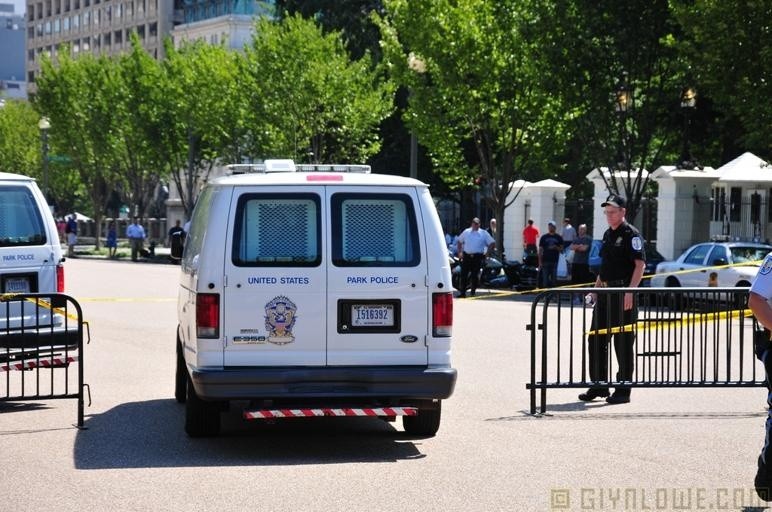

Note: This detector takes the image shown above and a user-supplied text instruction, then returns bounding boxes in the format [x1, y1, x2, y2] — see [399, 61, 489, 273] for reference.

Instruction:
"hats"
[601, 193, 626, 208]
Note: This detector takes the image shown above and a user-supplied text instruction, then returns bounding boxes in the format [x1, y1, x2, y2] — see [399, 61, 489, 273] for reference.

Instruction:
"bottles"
[584, 295, 597, 307]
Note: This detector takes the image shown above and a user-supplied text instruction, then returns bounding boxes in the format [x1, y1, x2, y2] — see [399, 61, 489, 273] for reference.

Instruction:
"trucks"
[170, 157, 458, 437]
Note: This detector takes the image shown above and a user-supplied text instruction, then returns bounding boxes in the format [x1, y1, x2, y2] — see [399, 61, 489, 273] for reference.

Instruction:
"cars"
[448, 252, 503, 291]
[650, 235, 772, 310]
[514, 238, 666, 298]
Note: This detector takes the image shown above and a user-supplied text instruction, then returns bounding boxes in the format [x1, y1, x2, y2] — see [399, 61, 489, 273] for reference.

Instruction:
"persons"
[56, 219, 68, 242]
[67, 213, 79, 258]
[125, 216, 146, 261]
[748, 252, 771, 501]
[106, 221, 118, 258]
[579, 194, 647, 405]
[443, 217, 592, 304]
[168, 219, 185, 264]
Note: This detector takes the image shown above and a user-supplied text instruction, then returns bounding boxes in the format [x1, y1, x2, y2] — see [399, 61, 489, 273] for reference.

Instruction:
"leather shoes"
[456, 293, 467, 298]
[606, 396, 630, 404]
[469, 291, 475, 297]
[755, 455, 772, 502]
[579, 389, 610, 401]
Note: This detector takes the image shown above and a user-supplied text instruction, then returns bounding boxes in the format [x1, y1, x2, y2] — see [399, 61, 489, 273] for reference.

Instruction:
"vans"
[1, 171, 84, 368]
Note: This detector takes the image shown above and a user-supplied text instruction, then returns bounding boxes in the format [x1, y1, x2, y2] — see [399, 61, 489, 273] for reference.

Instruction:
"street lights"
[38, 116, 52, 196]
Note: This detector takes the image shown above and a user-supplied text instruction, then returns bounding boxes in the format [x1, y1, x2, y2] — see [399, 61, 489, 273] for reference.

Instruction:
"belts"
[601, 279, 630, 287]
